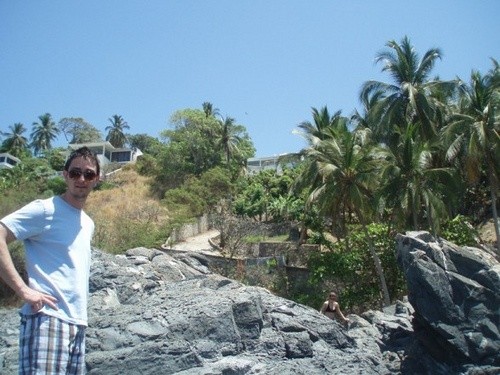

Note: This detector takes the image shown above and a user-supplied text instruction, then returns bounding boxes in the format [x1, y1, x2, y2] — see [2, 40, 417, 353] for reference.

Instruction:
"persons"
[319, 292, 350, 322]
[0, 145, 101, 375]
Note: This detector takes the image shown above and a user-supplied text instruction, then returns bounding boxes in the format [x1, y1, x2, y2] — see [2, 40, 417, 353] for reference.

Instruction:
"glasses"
[68, 169, 97, 181]
